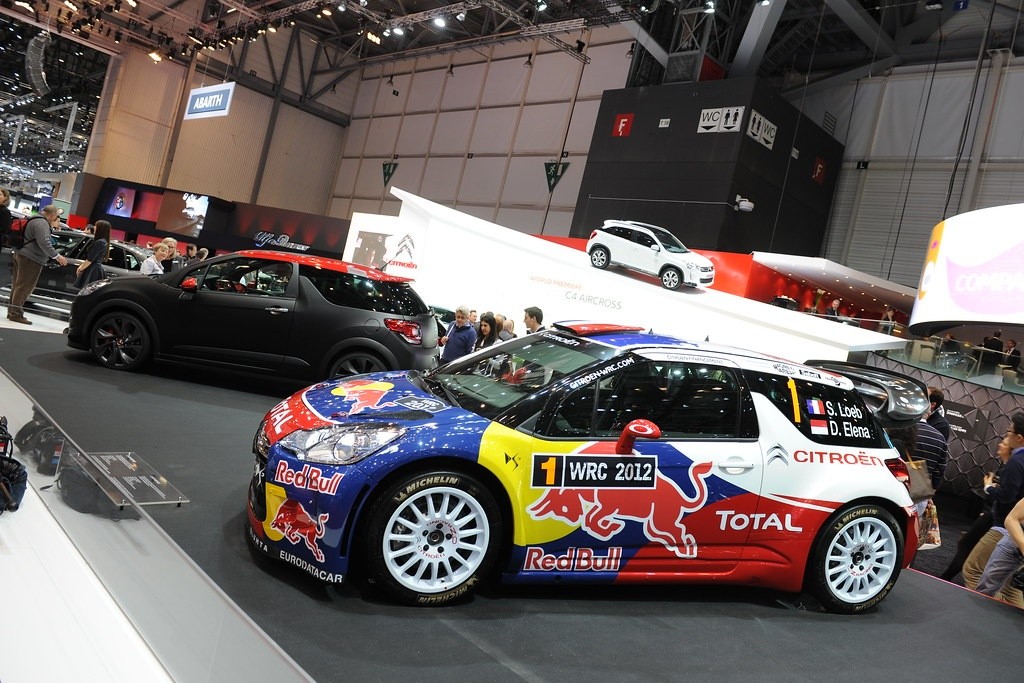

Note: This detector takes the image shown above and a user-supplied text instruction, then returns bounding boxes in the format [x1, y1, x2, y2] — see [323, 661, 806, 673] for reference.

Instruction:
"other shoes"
[7, 313, 32, 325]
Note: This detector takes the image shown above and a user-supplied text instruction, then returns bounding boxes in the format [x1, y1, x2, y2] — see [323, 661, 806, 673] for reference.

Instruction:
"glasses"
[1004, 429, 1017, 437]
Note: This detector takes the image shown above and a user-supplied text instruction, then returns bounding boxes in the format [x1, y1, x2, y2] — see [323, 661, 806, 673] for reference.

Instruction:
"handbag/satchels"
[905, 460, 935, 503]
[916, 501, 941, 552]
[439, 346, 445, 359]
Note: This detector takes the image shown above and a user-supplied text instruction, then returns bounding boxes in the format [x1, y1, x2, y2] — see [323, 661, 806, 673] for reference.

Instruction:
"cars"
[245, 321, 938, 614]
[61, 250, 439, 396]
[9, 209, 70, 235]
[7, 230, 155, 310]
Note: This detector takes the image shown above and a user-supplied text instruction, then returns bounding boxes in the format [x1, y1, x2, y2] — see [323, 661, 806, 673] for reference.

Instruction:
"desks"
[879, 323, 903, 334]
[837, 317, 860, 325]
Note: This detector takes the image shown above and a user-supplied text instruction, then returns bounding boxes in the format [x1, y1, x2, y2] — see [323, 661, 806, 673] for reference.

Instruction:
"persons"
[177, 249, 182, 256]
[184, 250, 207, 266]
[966, 337, 991, 372]
[523, 306, 546, 333]
[885, 386, 1024, 607]
[824, 299, 843, 322]
[480, 312, 493, 317]
[162, 237, 177, 273]
[130, 240, 134, 243]
[471, 314, 503, 354]
[1003, 339, 1021, 372]
[147, 242, 152, 248]
[84, 224, 94, 234]
[140, 243, 169, 274]
[480, 320, 514, 341]
[496, 316, 504, 333]
[52, 208, 64, 222]
[0, 186, 12, 258]
[469, 309, 479, 331]
[7, 205, 68, 325]
[200, 248, 209, 255]
[22, 206, 30, 214]
[975, 331, 1004, 375]
[72, 220, 111, 289]
[182, 244, 197, 262]
[939, 331, 962, 368]
[884, 307, 896, 339]
[440, 305, 477, 366]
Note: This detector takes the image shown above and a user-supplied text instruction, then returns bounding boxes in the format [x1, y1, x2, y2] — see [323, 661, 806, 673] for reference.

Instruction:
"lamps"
[0, 0, 770, 94]
[924, 0, 944, 11]
[735, 195, 754, 212]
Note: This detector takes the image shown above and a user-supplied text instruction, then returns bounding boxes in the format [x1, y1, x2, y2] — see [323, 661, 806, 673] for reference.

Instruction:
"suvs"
[584, 219, 717, 291]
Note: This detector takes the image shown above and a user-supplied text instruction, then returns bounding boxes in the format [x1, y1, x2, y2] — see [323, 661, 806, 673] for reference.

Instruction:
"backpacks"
[9, 215, 49, 250]
